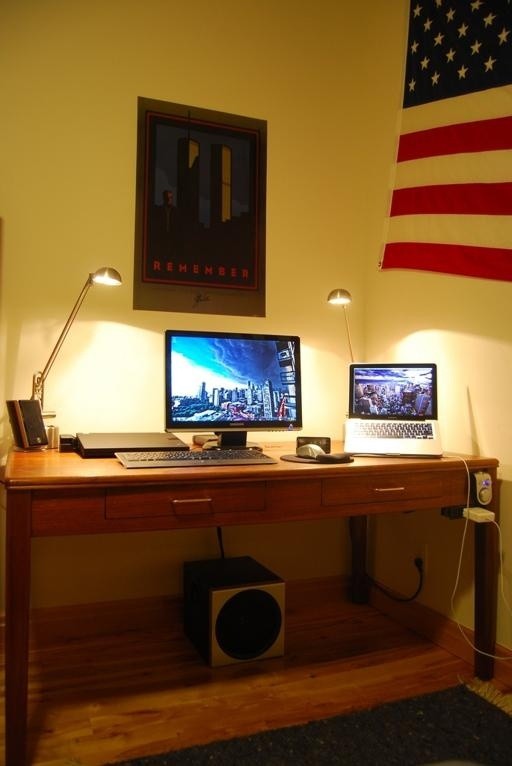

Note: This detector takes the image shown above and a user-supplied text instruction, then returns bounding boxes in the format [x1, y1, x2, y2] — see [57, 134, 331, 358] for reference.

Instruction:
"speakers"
[6, 398, 50, 452]
[183, 555, 287, 669]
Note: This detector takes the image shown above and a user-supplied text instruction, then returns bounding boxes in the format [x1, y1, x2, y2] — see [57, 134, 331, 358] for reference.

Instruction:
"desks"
[1, 437, 502, 766]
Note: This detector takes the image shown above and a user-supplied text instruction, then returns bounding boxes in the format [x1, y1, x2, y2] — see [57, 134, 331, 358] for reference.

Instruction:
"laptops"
[75, 431, 190, 459]
[343, 362, 445, 457]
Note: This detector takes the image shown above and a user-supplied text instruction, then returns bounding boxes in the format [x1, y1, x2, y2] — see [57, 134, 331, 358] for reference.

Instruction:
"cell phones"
[297, 437, 331, 453]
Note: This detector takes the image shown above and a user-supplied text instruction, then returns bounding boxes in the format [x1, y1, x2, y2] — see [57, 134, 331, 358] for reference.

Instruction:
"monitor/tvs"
[164, 329, 302, 451]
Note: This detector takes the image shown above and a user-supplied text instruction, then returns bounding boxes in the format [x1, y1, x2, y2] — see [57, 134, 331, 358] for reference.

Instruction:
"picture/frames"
[144, 111, 265, 294]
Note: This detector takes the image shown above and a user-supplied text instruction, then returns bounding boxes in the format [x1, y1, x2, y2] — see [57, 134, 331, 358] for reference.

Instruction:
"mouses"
[295, 444, 325, 459]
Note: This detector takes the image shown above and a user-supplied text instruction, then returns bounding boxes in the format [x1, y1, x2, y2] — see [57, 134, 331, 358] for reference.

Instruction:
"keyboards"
[114, 449, 279, 469]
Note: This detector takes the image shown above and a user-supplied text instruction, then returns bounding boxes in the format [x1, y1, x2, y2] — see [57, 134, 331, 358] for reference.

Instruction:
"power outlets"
[408, 543, 427, 577]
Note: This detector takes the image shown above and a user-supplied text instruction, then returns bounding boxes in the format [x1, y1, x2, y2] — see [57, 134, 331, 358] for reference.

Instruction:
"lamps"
[329, 287, 362, 364]
[38, 266, 127, 422]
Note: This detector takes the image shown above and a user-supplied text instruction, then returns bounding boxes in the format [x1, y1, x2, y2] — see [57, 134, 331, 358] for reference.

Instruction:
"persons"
[153, 188, 178, 243]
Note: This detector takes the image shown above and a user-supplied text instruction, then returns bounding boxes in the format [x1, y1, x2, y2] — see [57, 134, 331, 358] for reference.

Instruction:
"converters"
[463, 507, 497, 527]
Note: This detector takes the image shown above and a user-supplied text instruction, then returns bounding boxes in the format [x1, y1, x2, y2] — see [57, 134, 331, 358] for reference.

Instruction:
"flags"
[380, 0, 512, 285]
